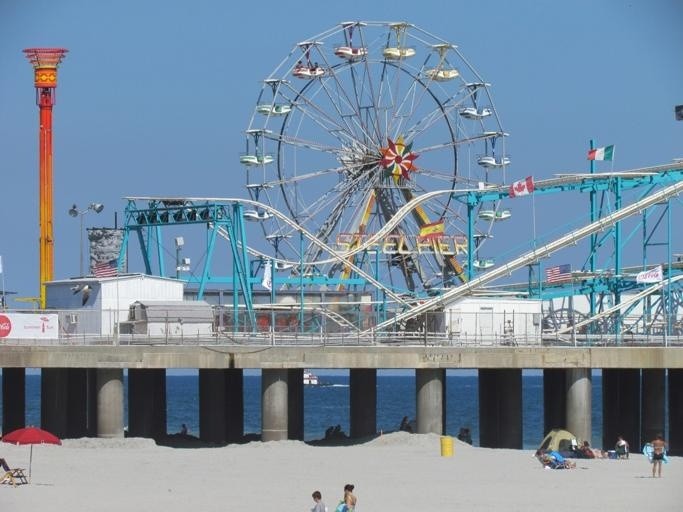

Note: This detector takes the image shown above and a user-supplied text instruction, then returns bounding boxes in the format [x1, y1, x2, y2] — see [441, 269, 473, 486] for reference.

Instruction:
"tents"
[536, 427, 579, 458]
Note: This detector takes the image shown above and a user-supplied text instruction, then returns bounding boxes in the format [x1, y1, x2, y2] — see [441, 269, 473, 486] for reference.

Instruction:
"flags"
[508, 174, 533, 198]
[545, 264, 572, 283]
[95, 258, 118, 278]
[261, 257, 272, 293]
[636, 264, 662, 284]
[418, 218, 445, 241]
[587, 145, 614, 161]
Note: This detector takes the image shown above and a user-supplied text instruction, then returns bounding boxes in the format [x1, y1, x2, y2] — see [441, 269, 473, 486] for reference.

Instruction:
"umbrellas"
[2, 425, 62, 482]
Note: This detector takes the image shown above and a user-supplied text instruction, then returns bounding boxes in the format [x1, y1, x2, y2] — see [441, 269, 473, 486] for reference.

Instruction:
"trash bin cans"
[440, 436, 454, 457]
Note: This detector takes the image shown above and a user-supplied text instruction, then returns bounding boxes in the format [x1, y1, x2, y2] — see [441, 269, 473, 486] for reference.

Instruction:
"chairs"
[0, 458, 29, 484]
[618, 444, 629, 460]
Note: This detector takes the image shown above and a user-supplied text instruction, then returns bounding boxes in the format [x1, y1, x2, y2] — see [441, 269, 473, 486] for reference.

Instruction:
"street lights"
[69, 201, 103, 279]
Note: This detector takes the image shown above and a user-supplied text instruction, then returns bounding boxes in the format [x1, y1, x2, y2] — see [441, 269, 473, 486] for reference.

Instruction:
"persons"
[400, 416, 411, 431]
[181, 424, 187, 435]
[324, 425, 350, 440]
[615, 435, 629, 458]
[457, 427, 473, 445]
[647, 433, 668, 478]
[311, 491, 326, 512]
[0, 457, 10, 472]
[344, 484, 357, 512]
